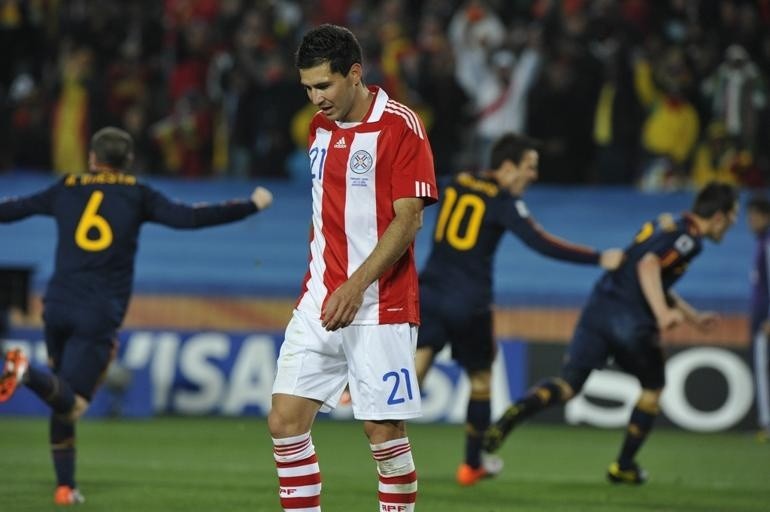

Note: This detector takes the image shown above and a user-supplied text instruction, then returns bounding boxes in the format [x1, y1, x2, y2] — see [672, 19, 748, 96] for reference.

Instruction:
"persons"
[483, 175, 737, 487]
[0, 127, 274, 505]
[411, 131, 624, 488]
[745, 197, 769, 430]
[268, 20, 442, 512]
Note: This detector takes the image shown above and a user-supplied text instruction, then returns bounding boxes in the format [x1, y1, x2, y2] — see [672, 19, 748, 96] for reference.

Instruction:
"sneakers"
[54, 483, 87, 506]
[605, 459, 651, 484]
[456, 448, 504, 486]
[2, 347, 31, 404]
